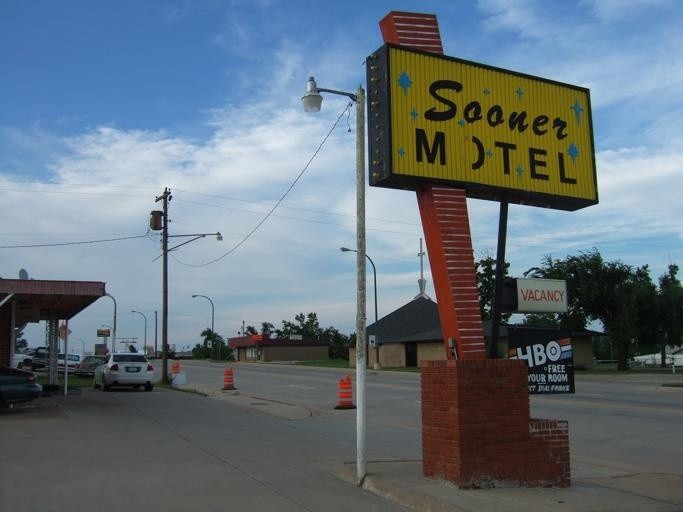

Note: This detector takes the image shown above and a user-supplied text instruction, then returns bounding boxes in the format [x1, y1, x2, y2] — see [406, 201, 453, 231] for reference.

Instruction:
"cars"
[12, 347, 105, 377]
[92, 352, 154, 392]
[0, 362, 42, 415]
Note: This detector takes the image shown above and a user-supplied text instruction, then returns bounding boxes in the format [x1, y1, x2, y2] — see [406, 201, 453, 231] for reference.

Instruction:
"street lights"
[99, 324, 113, 353]
[161, 231, 222, 385]
[103, 292, 116, 352]
[191, 295, 214, 360]
[130, 311, 146, 354]
[340, 247, 381, 369]
[75, 338, 84, 358]
[300, 76, 368, 485]
